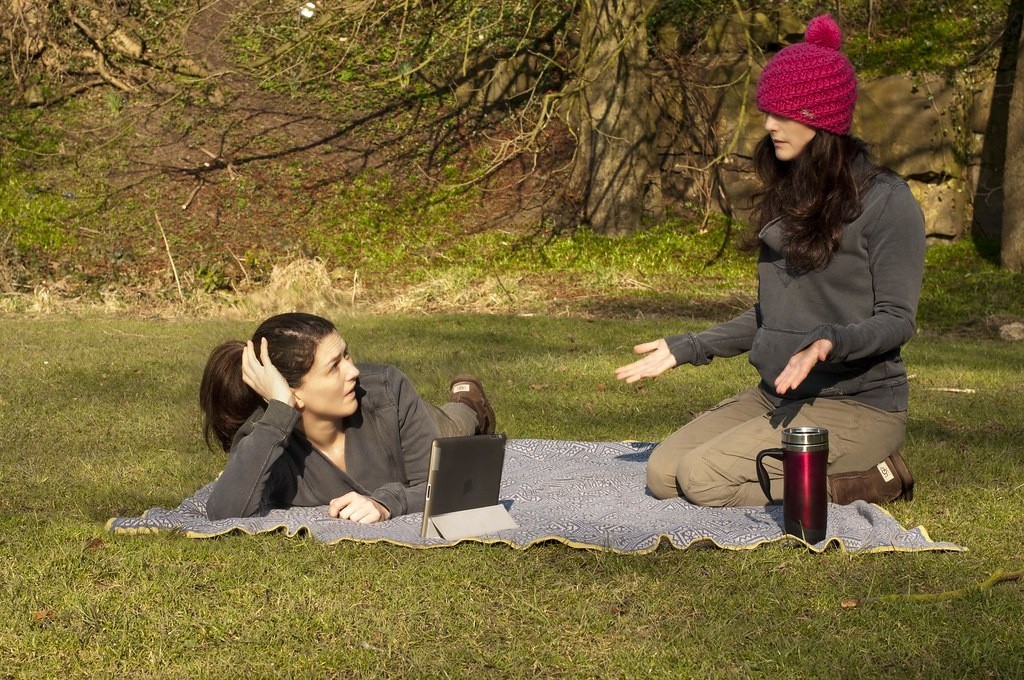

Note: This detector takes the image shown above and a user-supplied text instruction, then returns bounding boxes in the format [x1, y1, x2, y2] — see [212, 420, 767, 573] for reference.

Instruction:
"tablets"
[421, 433, 508, 538]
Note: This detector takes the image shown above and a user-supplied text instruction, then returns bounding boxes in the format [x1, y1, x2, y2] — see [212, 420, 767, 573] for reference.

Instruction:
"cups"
[756, 427, 830, 543]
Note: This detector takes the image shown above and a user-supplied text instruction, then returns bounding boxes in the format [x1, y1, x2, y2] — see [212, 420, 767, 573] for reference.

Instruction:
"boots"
[449, 375, 497, 436]
[828, 448, 913, 505]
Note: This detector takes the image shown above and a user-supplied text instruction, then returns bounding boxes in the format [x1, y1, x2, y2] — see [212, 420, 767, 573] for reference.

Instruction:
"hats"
[755, 15, 858, 135]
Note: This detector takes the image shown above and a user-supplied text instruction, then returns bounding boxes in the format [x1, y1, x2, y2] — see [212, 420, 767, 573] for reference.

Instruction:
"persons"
[616, 16, 927, 506]
[201, 313, 495, 523]
